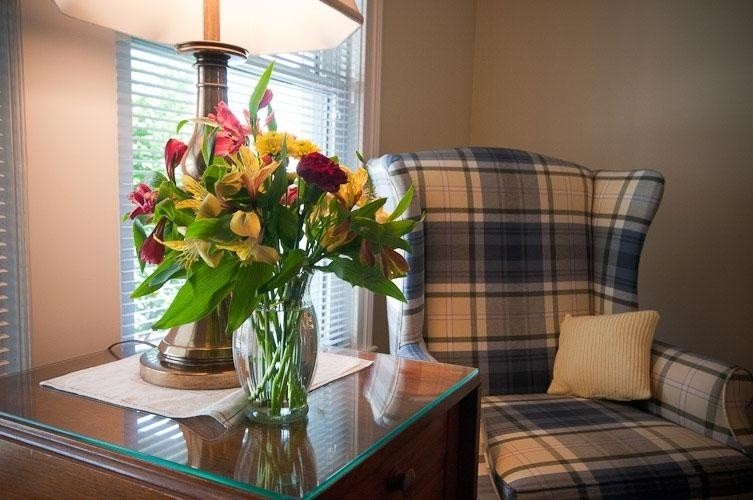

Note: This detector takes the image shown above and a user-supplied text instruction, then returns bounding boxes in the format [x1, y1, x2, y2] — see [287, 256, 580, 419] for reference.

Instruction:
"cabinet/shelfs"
[1, 333, 484, 499]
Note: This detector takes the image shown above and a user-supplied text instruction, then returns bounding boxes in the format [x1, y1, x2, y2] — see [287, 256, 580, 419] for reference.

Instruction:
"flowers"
[118, 54, 427, 419]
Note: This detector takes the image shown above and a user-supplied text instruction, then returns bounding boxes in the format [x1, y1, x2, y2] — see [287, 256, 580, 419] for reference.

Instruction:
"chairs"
[360, 140, 752, 500]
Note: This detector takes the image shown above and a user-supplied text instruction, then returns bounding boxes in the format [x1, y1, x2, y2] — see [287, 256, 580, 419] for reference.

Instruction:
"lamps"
[41, 1, 372, 398]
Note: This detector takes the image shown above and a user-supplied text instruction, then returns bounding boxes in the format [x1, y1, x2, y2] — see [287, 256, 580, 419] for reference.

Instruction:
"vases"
[222, 289, 328, 429]
[232, 420, 320, 499]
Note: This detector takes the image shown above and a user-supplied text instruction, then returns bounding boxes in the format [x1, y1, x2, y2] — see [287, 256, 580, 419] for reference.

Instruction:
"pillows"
[545, 308, 659, 401]
